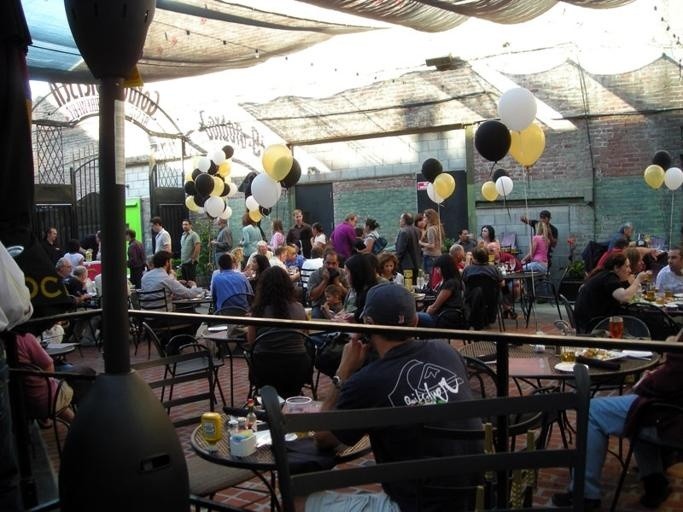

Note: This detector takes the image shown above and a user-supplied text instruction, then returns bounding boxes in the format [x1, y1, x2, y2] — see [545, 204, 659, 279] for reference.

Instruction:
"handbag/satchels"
[372, 238, 387, 254]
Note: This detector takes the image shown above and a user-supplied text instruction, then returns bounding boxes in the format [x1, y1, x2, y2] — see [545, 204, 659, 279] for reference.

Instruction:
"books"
[476, 86, 547, 205]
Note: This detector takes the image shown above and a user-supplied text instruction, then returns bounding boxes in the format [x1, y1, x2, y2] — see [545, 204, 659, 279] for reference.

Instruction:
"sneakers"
[552, 488, 602, 512]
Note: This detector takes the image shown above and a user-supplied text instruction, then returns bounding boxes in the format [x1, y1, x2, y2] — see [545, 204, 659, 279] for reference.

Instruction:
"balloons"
[421, 157, 456, 203]
[180, 141, 234, 220]
[643, 147, 683, 192]
[246, 141, 303, 223]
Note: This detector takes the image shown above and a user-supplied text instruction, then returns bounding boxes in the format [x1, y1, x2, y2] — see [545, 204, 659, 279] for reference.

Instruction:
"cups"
[304, 308, 312, 321]
[510, 247, 516, 254]
[634, 284, 673, 305]
[498, 257, 516, 275]
[559, 329, 576, 365]
[283, 396, 313, 441]
[609, 315, 622, 338]
[644, 233, 649, 242]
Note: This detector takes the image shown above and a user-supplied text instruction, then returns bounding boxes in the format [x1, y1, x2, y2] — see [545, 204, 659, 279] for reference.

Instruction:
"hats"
[359, 282, 416, 324]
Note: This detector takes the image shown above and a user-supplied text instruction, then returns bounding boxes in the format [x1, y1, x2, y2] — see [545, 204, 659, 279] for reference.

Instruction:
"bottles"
[40, 339, 48, 350]
[245, 399, 256, 433]
[534, 331, 545, 354]
[201, 411, 223, 452]
[237, 417, 246, 431]
[85, 250, 91, 261]
[416, 269, 424, 289]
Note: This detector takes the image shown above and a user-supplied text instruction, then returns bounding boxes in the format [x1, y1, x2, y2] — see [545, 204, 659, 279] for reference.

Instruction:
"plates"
[664, 303, 678, 309]
[576, 347, 628, 363]
[553, 362, 588, 372]
[673, 294, 682, 298]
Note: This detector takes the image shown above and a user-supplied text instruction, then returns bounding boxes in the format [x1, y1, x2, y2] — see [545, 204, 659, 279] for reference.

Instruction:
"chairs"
[258, 363, 591, 511]
[3, 362, 95, 508]
[208, 307, 250, 406]
[164, 342, 277, 511]
[604, 390, 681, 510]
[140, 321, 228, 407]
[0, 232, 682, 360]
[246, 329, 318, 402]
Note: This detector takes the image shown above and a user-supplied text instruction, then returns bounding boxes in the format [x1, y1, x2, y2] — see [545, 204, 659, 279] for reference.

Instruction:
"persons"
[573, 221, 683, 338]
[0, 238, 34, 492]
[551, 325, 683, 512]
[287, 283, 492, 511]
[13, 325, 76, 428]
[36, 209, 559, 397]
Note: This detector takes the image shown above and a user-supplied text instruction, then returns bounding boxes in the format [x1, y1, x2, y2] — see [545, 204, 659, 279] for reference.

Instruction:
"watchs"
[332, 374, 343, 388]
